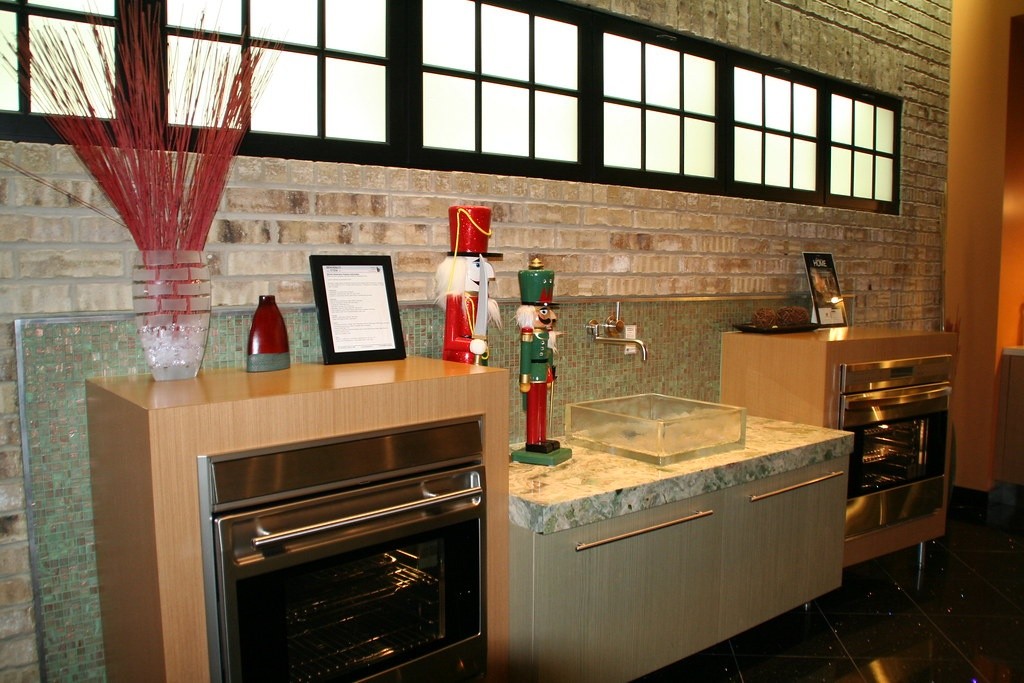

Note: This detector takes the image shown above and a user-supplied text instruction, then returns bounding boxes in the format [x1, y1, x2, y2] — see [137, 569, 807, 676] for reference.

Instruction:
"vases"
[132, 252, 212, 383]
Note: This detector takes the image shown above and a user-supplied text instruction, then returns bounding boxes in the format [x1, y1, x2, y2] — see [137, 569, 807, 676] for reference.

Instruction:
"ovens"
[720, 329, 958, 569]
[197, 417, 490, 683]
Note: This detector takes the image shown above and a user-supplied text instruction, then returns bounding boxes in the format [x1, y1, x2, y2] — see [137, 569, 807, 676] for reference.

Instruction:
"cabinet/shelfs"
[508, 431, 855, 683]
